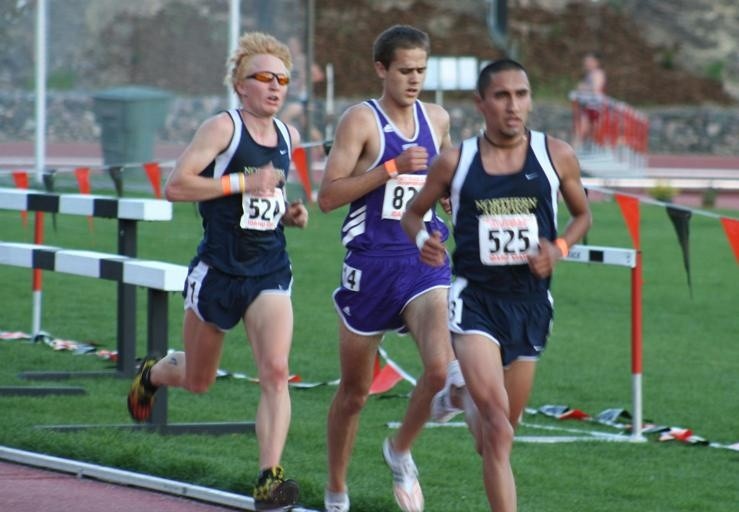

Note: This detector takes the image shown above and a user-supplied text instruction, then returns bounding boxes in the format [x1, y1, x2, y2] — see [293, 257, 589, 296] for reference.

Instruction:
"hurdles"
[383, 243, 647, 445]
[3, 241, 258, 436]
[1, 188, 176, 382]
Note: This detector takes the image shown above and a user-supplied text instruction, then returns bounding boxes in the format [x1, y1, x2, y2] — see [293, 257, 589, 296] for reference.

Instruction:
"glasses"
[240, 71, 292, 86]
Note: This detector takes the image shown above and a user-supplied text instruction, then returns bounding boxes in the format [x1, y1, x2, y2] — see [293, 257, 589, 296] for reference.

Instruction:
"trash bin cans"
[90, 85, 176, 186]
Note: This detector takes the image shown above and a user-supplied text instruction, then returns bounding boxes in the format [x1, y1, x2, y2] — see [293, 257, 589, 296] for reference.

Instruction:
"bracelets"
[555, 239, 573, 262]
[384, 159, 397, 178]
[411, 228, 432, 255]
[220, 171, 246, 195]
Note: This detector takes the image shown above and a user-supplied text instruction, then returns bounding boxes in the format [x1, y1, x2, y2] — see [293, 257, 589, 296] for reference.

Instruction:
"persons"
[316, 23, 453, 512]
[572, 53, 608, 155]
[398, 58, 593, 510]
[282, 37, 324, 159]
[126, 29, 309, 512]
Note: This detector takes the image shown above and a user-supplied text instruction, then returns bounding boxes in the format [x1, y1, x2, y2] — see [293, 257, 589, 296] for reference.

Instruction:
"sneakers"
[126, 350, 158, 423]
[253, 465, 300, 512]
[381, 434, 425, 511]
[428, 358, 467, 424]
[323, 483, 350, 512]
